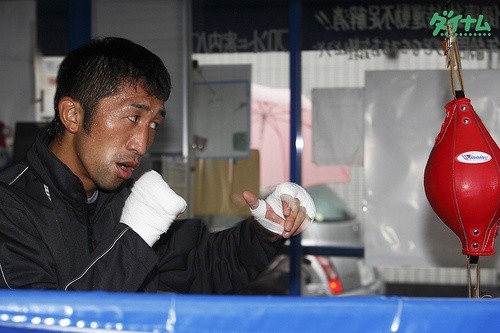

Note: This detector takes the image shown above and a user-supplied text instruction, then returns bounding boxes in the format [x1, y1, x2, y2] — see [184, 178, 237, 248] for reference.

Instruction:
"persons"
[0, 34, 318, 296]
